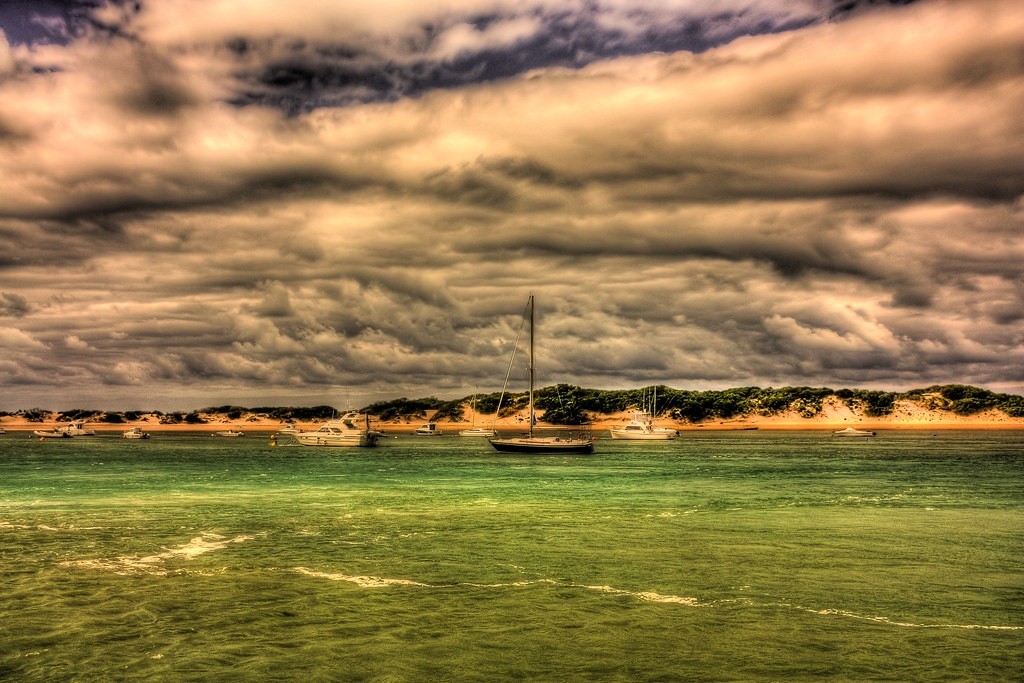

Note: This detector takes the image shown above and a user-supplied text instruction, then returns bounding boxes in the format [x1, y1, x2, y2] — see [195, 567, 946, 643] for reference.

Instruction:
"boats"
[278, 424, 304, 435]
[216, 430, 244, 437]
[296, 412, 387, 449]
[32, 427, 73, 438]
[416, 422, 444, 439]
[61, 422, 97, 436]
[835, 427, 876, 439]
[122, 427, 151, 439]
[607, 384, 679, 441]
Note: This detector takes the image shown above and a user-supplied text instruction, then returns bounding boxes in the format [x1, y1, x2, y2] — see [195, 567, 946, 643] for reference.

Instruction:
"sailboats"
[487, 291, 595, 454]
[458, 384, 500, 438]
